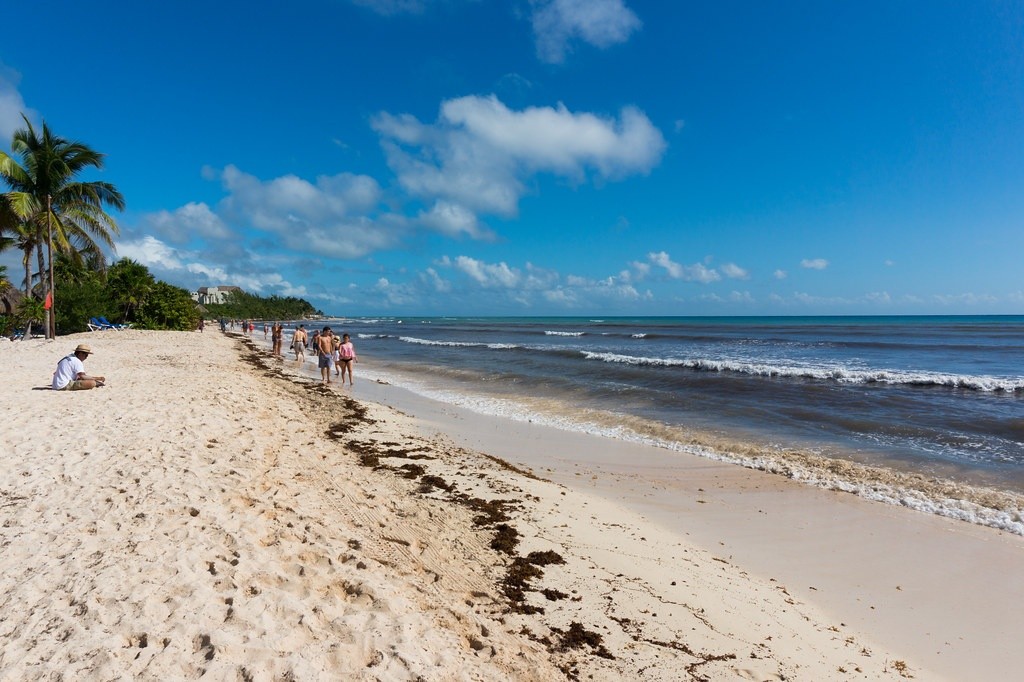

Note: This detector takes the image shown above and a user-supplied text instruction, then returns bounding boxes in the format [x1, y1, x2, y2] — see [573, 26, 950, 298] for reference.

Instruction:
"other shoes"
[95, 380, 105, 387]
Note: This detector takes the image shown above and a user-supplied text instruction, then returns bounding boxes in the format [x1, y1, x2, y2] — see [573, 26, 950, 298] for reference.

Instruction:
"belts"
[295, 341, 304, 342]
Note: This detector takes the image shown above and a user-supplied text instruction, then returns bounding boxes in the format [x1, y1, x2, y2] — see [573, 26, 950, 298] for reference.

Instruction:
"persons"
[199, 319, 205, 333]
[51, 344, 106, 391]
[231, 318, 234, 327]
[242, 320, 252, 334]
[271, 323, 283, 355]
[332, 336, 341, 376]
[288, 322, 290, 326]
[220, 318, 228, 331]
[329, 331, 334, 338]
[291, 326, 307, 362]
[300, 324, 307, 337]
[310, 330, 320, 356]
[317, 327, 332, 383]
[264, 323, 268, 340]
[338, 334, 358, 385]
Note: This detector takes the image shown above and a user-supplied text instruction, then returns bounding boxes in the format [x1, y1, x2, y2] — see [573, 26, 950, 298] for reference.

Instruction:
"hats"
[332, 336, 341, 342]
[73, 344, 93, 354]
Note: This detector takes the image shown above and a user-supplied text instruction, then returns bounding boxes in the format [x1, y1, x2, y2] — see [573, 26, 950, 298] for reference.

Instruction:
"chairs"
[99, 317, 134, 332]
[87, 317, 120, 335]
